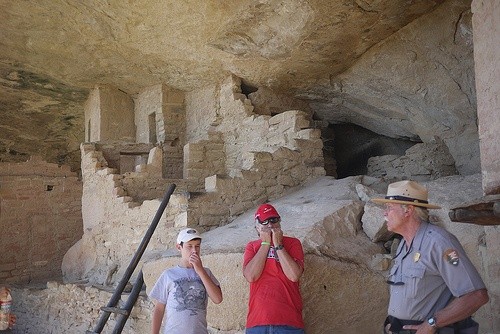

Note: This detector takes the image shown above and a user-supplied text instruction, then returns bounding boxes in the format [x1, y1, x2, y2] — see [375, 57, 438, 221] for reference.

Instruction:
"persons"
[0, 285, 16, 334]
[148, 228, 223, 334]
[242, 203, 305, 334]
[372, 181, 489, 334]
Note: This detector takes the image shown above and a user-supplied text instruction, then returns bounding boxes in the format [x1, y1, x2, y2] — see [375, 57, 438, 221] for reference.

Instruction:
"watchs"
[427, 316, 438, 331]
[275, 245, 284, 251]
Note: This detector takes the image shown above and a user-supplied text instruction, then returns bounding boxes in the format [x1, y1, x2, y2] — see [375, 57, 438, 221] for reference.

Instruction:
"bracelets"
[261, 241, 271, 245]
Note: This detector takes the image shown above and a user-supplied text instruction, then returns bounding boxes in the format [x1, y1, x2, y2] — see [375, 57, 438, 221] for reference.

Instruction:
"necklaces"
[387, 236, 415, 285]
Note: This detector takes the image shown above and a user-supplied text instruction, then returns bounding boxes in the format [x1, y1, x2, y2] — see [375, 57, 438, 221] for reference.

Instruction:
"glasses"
[386, 260, 404, 286]
[257, 217, 278, 225]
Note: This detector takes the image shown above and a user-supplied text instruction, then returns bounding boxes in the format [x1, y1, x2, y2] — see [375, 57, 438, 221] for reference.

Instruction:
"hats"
[371, 180, 442, 209]
[255, 204, 280, 221]
[177, 228, 202, 244]
